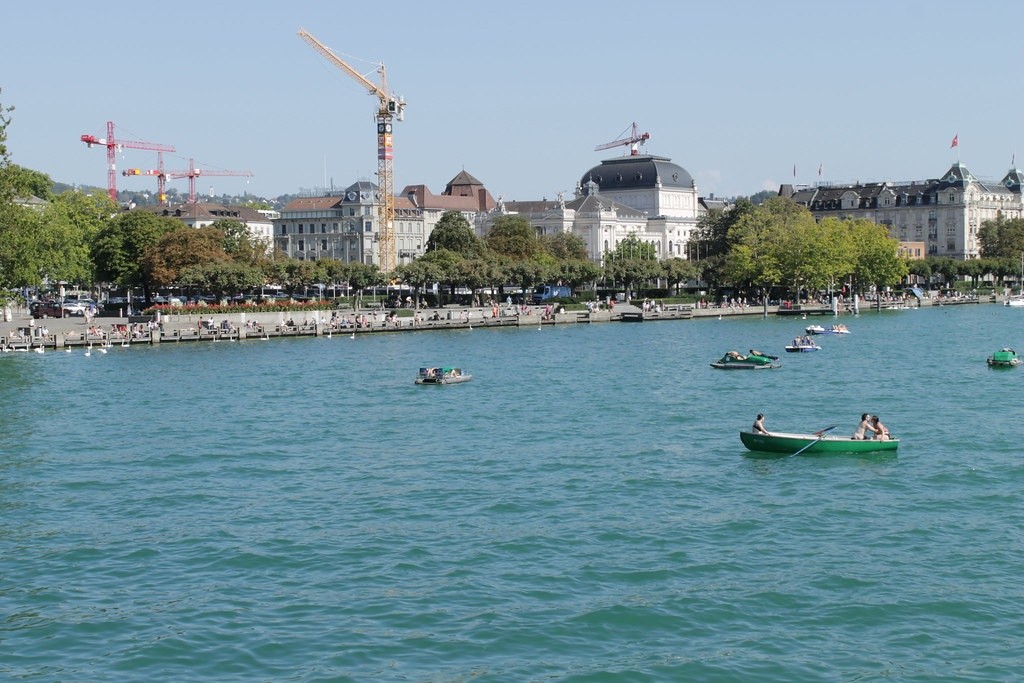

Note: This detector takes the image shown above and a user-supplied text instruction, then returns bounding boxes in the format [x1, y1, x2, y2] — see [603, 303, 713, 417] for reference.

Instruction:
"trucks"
[531, 283, 571, 305]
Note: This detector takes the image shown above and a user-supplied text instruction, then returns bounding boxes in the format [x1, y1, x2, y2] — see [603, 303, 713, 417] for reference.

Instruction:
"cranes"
[298, 27, 408, 290]
[172, 156, 256, 204]
[81, 120, 177, 207]
[119, 151, 205, 207]
[593, 121, 649, 158]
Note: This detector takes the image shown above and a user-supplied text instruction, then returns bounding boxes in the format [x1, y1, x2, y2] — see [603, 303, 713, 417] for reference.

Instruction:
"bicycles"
[394, 299, 403, 308]
[404, 301, 415, 311]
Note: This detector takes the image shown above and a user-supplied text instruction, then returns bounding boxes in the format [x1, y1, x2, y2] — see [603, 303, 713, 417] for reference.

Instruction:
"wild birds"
[0, 343, 45, 354]
[940, 303, 943, 307]
[718, 315, 722, 320]
[120, 339, 130, 348]
[229, 336, 235, 342]
[212, 335, 221, 343]
[327, 331, 333, 339]
[65, 346, 72, 353]
[259, 334, 270, 341]
[537, 326, 542, 331]
[802, 314, 807, 320]
[83, 340, 114, 357]
[349, 333, 356, 340]
[469, 324, 474, 330]
[885, 304, 918, 310]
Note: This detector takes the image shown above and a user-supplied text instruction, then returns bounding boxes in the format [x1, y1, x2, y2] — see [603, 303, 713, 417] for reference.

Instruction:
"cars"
[55, 283, 323, 317]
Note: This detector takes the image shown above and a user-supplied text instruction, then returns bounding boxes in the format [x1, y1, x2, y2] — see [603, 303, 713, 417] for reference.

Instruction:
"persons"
[753, 413, 770, 435]
[749, 350, 778, 360]
[8, 269, 1021, 349]
[871, 416, 889, 441]
[853, 413, 879, 440]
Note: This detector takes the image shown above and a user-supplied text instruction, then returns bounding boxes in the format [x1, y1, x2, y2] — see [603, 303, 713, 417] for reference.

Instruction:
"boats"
[805, 325, 851, 336]
[987, 347, 1023, 367]
[415, 364, 472, 385]
[710, 353, 782, 371]
[740, 429, 901, 453]
[1003, 294, 1024, 306]
[785, 339, 822, 353]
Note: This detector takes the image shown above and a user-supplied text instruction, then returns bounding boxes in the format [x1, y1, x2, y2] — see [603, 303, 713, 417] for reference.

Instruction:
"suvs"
[29, 301, 71, 320]
